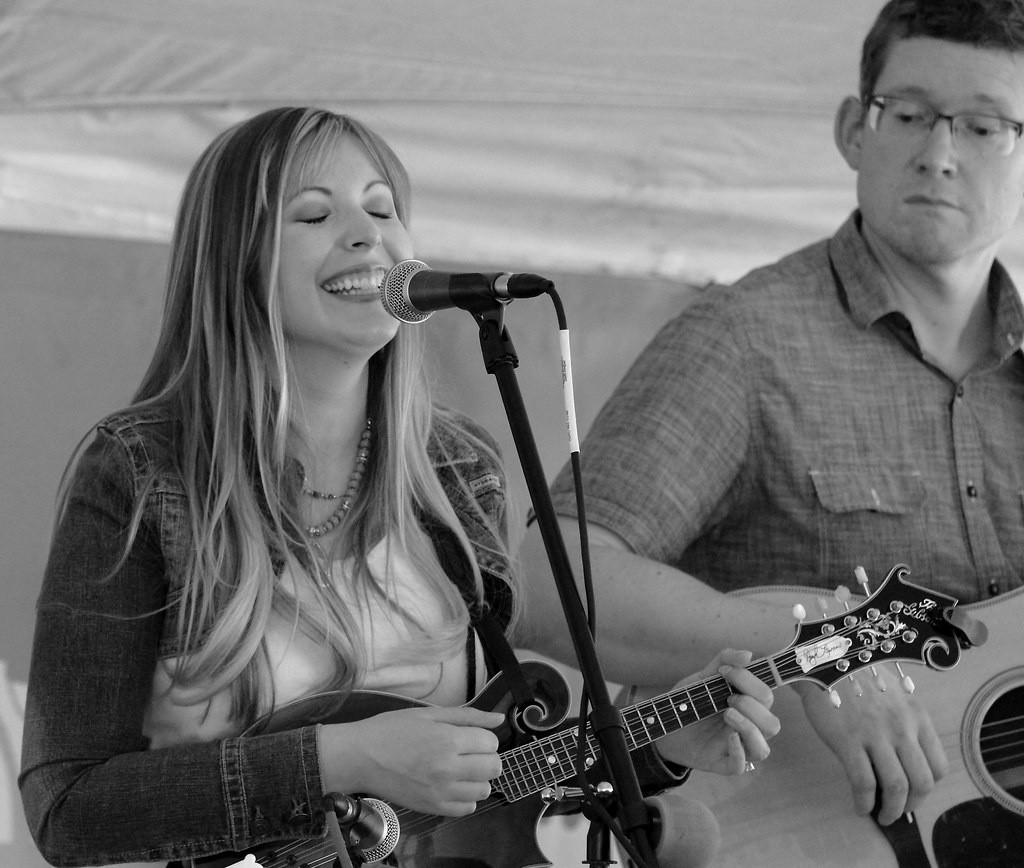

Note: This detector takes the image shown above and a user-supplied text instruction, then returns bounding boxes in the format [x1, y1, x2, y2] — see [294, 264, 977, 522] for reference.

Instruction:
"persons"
[518, 0, 1024, 868]
[17, 105, 778, 868]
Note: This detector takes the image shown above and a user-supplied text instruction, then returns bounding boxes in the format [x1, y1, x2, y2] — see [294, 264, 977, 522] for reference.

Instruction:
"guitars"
[167, 568, 987, 868]
[605, 583, 1023, 867]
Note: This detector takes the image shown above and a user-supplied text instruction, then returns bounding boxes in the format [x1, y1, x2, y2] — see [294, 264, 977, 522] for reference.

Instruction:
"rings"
[745, 760, 756, 778]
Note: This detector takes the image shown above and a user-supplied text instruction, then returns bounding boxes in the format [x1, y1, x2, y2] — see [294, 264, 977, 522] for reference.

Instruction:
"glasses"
[860, 93, 1024, 162]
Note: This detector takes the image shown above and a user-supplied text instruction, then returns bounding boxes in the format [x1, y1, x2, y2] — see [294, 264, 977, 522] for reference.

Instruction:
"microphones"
[380, 259, 555, 324]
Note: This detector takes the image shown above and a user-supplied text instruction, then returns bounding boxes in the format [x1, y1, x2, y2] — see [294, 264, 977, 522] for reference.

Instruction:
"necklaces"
[298, 400, 377, 537]
